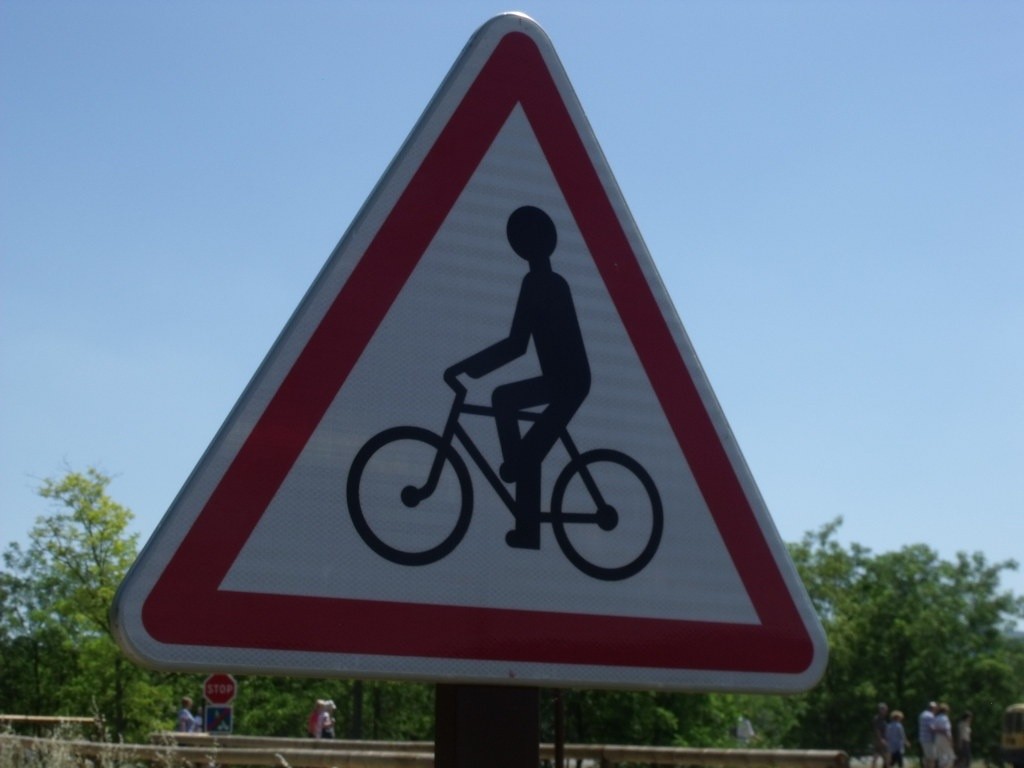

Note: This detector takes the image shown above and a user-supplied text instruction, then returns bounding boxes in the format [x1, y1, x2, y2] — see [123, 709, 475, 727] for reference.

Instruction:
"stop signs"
[204, 674, 237, 704]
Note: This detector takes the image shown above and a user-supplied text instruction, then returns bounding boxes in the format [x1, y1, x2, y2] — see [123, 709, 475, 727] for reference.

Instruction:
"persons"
[918, 701, 972, 768]
[736, 712, 761, 743]
[308, 699, 336, 738]
[871, 702, 911, 768]
[176, 696, 203, 732]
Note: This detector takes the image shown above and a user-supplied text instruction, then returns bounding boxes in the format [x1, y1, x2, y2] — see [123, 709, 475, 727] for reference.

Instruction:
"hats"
[324, 700, 337, 710]
